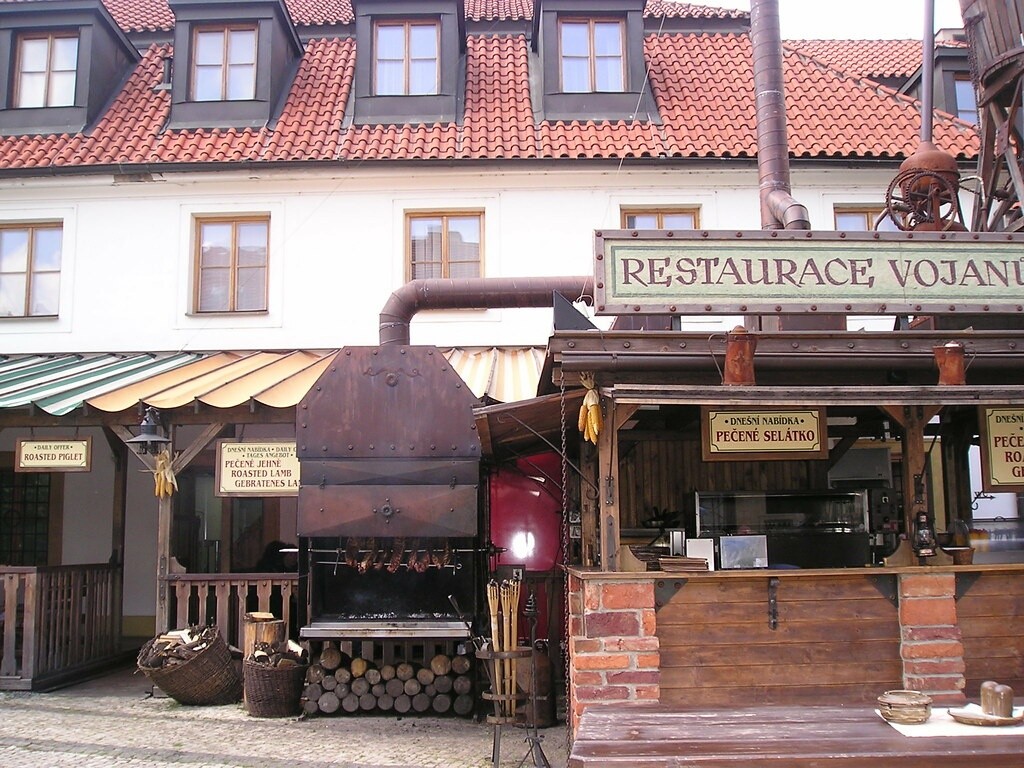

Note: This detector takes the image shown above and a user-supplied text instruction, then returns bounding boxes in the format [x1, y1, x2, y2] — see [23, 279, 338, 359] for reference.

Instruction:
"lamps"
[124, 406, 171, 458]
[912, 507, 938, 557]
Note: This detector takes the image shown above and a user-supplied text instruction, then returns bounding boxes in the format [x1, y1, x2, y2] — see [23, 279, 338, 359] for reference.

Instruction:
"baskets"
[138, 624, 243, 706]
[243, 654, 308, 717]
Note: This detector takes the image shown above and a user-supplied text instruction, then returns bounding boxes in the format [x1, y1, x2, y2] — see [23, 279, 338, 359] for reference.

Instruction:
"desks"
[566, 697, 1024, 768]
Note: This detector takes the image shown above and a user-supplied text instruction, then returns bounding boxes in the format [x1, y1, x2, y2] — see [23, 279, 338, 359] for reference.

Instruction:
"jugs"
[708, 325, 760, 386]
[932, 339, 977, 386]
